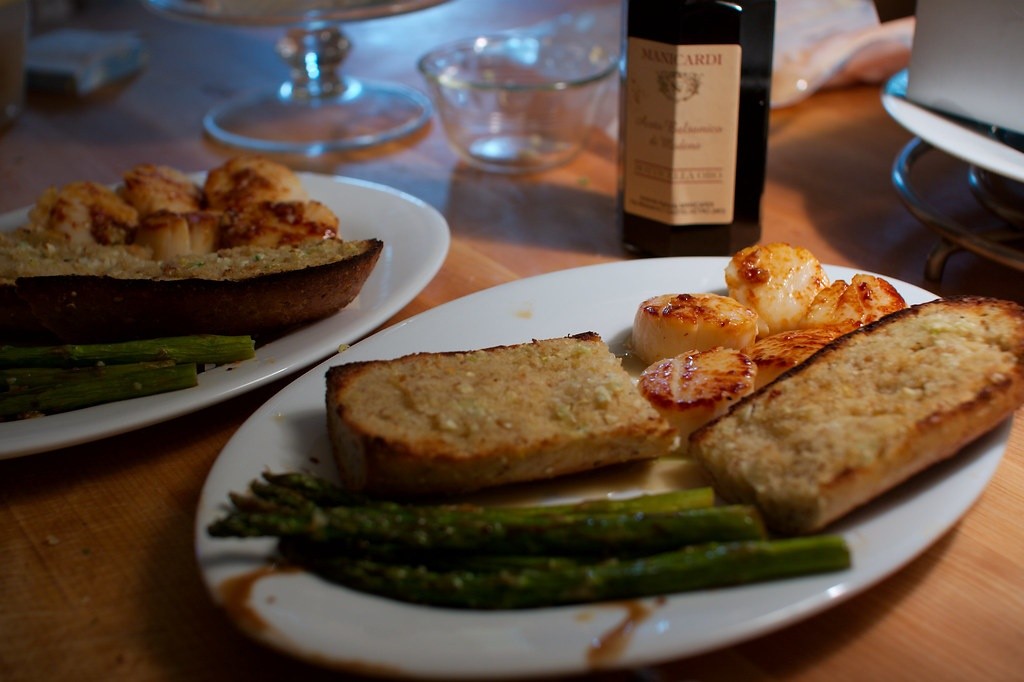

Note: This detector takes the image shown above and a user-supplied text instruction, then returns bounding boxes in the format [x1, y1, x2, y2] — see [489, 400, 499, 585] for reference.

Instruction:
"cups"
[0, 0, 28, 135]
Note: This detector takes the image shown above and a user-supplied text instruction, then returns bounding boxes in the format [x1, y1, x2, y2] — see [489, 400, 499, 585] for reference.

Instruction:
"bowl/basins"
[416, 32, 624, 174]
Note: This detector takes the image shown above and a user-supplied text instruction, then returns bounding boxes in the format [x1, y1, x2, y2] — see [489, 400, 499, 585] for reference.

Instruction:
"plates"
[0, 170, 450, 461]
[194, 256, 1015, 678]
[878, 67, 1024, 184]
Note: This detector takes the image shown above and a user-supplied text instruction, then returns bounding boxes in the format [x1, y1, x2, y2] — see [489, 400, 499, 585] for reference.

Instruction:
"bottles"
[626, 0, 777, 260]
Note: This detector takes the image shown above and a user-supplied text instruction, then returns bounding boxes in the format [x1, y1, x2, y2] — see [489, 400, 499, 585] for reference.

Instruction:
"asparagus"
[205, 470, 851, 612]
[0, 333, 256, 422]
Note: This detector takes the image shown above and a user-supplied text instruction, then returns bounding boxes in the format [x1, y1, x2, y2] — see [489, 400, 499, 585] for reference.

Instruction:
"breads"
[324, 331, 681, 497]
[1, 233, 383, 335]
[679, 290, 1023, 535]
